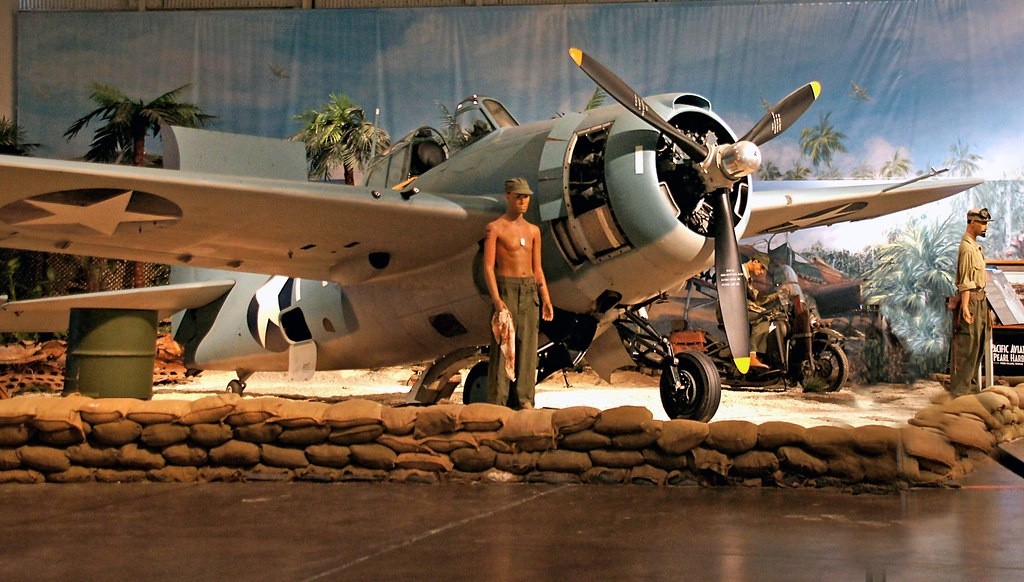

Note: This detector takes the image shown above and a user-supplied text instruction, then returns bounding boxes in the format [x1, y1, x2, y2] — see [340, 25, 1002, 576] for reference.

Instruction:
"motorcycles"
[704, 264, 848, 392]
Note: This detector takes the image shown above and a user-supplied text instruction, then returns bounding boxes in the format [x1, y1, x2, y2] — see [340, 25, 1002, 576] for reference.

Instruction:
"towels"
[491, 307, 520, 382]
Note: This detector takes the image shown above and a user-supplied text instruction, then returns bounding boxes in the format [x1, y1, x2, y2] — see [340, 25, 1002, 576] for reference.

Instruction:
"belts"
[969, 291, 987, 300]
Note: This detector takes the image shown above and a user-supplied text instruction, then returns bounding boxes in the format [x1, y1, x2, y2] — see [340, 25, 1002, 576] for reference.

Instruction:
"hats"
[504, 177, 534, 195]
[966, 208, 995, 222]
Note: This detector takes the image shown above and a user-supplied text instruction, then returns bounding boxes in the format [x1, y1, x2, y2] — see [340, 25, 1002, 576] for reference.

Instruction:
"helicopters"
[1, 46, 984, 423]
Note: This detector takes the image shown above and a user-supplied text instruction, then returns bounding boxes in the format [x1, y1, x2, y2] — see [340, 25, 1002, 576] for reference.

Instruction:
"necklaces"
[520, 237, 527, 247]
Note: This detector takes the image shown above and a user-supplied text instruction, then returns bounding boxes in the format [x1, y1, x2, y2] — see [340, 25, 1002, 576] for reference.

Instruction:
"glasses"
[968, 208, 992, 219]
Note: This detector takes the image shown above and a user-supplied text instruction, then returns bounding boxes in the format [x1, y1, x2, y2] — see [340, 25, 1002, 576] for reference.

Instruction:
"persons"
[481, 177, 554, 411]
[948, 207, 997, 398]
[716, 251, 773, 373]
